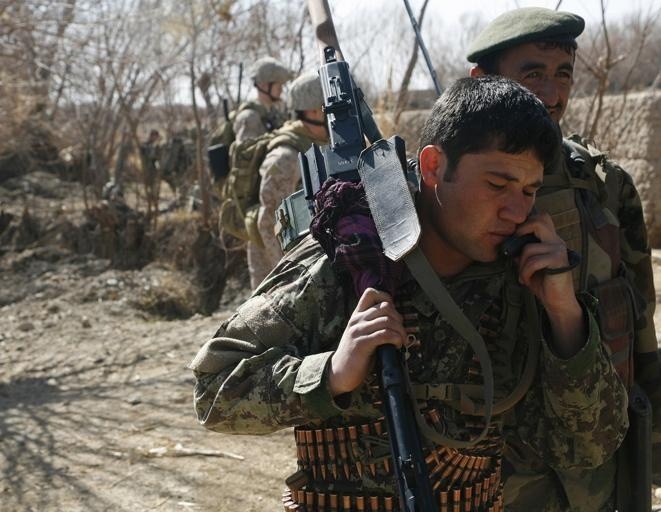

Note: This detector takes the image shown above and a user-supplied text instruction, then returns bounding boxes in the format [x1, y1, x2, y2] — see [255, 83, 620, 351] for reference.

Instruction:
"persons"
[140, 130, 160, 179]
[190, 75, 630, 512]
[208, 57, 329, 292]
[465, 7, 661, 512]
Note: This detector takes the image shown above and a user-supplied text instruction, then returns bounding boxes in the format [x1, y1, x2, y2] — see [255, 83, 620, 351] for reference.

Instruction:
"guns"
[298, 0, 433, 512]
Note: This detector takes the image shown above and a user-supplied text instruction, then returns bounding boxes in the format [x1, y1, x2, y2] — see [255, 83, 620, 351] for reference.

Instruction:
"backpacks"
[215, 131, 314, 250]
[206, 99, 272, 201]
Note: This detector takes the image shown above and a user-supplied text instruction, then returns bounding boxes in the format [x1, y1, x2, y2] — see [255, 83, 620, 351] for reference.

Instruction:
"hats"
[459, 4, 587, 64]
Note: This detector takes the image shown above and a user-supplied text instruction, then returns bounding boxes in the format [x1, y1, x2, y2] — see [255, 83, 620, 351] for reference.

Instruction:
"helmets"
[248, 59, 291, 86]
[286, 69, 325, 112]
